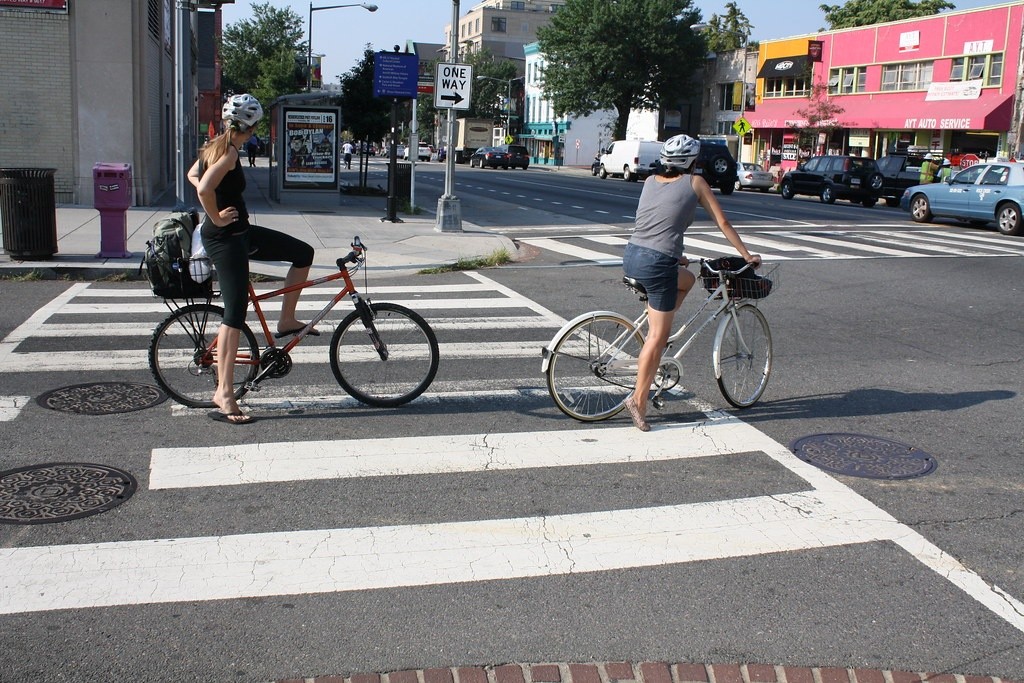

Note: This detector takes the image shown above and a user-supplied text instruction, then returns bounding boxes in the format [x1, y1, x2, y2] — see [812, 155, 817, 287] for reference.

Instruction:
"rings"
[234, 219, 236, 222]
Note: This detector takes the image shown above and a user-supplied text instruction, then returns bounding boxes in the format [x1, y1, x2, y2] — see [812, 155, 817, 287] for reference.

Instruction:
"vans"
[598, 139, 666, 182]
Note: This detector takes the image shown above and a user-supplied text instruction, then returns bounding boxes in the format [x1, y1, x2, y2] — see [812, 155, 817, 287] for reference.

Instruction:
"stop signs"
[959, 154, 981, 173]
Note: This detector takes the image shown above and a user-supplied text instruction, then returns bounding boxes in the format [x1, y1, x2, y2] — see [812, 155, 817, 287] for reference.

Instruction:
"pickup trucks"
[870, 154, 949, 208]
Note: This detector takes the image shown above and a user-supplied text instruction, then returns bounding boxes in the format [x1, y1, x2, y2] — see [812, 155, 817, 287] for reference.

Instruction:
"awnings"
[756, 54, 813, 80]
[737, 94, 1013, 132]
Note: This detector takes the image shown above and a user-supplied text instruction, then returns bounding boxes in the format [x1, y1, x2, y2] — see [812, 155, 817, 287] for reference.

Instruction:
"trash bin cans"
[0, 168, 59, 261]
[386, 163, 418, 209]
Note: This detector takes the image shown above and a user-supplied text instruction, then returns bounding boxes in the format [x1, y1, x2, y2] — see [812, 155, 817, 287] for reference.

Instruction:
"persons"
[342, 141, 353, 170]
[920, 153, 939, 184]
[288, 135, 316, 169]
[622, 134, 762, 432]
[246, 135, 257, 167]
[937, 159, 955, 182]
[188, 93, 320, 423]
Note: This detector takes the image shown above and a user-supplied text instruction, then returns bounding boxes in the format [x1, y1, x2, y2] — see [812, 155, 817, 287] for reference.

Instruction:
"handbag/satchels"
[247, 144, 258, 152]
[188, 223, 211, 284]
[701, 257, 772, 300]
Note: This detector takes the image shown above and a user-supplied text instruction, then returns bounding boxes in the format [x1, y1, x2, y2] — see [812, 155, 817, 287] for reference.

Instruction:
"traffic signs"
[434, 62, 474, 113]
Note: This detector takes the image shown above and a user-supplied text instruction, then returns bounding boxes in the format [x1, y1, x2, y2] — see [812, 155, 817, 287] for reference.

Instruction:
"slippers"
[208, 412, 254, 424]
[274, 324, 321, 336]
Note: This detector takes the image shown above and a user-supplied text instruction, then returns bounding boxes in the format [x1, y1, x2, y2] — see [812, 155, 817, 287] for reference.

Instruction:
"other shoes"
[252, 163, 256, 167]
[348, 166, 350, 169]
[345, 165, 347, 169]
[623, 398, 650, 432]
[250, 163, 252, 167]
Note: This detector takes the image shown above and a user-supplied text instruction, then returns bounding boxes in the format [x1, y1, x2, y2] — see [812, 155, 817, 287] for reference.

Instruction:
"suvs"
[499, 144, 532, 170]
[403, 142, 432, 161]
[654, 142, 738, 195]
[779, 155, 883, 207]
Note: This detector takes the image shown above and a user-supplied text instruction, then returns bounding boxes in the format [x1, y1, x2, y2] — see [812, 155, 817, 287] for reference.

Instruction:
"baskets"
[699, 263, 781, 301]
[143, 258, 212, 297]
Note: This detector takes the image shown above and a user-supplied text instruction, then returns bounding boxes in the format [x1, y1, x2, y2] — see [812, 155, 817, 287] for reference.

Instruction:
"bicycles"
[145, 235, 440, 408]
[540, 254, 775, 420]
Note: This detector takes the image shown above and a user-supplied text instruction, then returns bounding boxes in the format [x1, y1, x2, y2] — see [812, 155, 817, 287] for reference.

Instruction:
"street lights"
[691, 22, 752, 166]
[307, 1, 381, 92]
[476, 74, 525, 143]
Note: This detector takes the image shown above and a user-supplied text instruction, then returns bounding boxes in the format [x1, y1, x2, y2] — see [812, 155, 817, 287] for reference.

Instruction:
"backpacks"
[138, 208, 198, 296]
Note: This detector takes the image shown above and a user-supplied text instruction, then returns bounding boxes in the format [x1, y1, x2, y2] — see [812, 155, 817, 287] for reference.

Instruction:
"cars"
[589, 148, 604, 175]
[901, 155, 1024, 237]
[736, 162, 777, 192]
[356, 144, 376, 156]
[386, 145, 405, 159]
[469, 146, 510, 171]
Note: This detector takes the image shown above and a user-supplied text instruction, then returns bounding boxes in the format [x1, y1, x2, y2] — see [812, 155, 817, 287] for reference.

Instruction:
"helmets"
[659, 134, 700, 168]
[221, 94, 263, 127]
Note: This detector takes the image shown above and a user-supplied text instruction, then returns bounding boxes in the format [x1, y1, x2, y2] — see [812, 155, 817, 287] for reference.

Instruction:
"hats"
[924, 153, 932, 160]
[943, 159, 951, 165]
